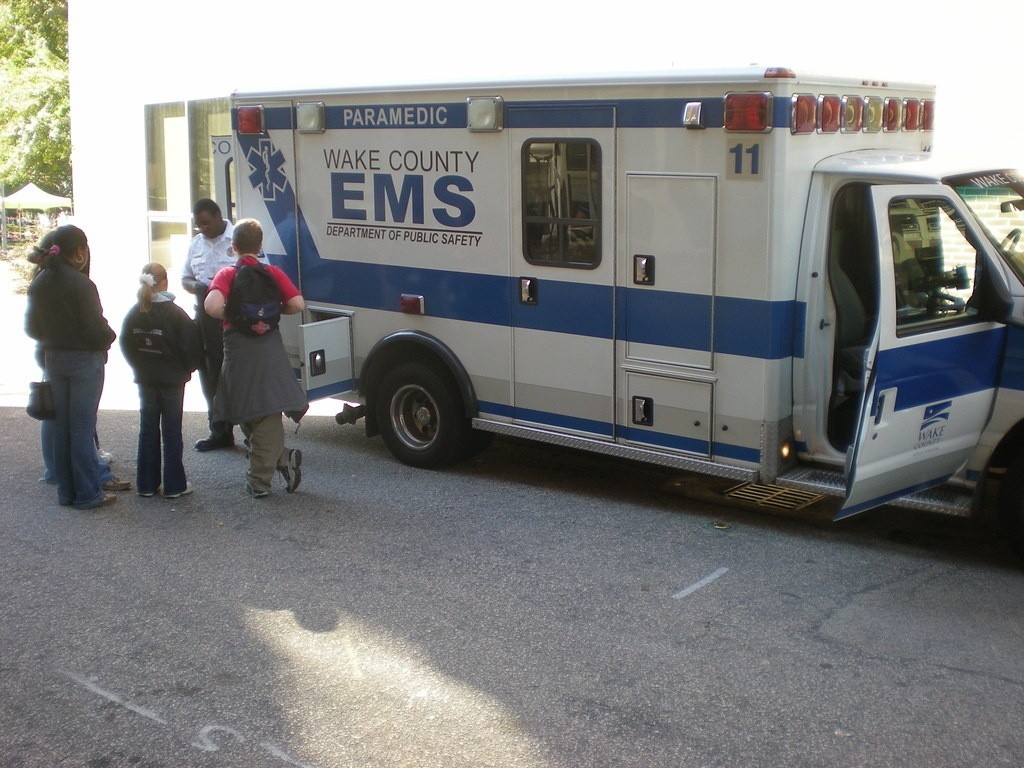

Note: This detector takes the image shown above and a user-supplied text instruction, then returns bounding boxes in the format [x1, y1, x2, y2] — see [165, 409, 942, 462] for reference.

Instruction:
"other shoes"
[246, 482, 268, 498]
[97, 448, 113, 463]
[101, 477, 131, 491]
[137, 485, 161, 496]
[194, 438, 234, 450]
[165, 481, 192, 498]
[96, 494, 117, 507]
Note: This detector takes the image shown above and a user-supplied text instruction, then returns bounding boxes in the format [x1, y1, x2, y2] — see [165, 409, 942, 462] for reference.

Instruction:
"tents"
[0, 183, 72, 240]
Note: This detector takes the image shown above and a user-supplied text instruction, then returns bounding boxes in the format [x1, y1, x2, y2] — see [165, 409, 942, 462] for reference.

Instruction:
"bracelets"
[193, 281, 199, 290]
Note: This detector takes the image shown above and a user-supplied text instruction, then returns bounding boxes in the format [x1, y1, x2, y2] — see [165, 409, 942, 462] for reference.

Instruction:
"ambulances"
[210, 64, 1024, 574]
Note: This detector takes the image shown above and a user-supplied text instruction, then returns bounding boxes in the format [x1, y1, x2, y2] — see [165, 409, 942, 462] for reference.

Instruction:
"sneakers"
[276, 448, 302, 493]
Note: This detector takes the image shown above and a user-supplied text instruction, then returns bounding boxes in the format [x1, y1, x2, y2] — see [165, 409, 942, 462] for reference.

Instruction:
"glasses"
[193, 217, 215, 234]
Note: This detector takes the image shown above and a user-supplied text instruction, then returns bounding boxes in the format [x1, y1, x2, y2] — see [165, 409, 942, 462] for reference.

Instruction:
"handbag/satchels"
[26, 381, 55, 421]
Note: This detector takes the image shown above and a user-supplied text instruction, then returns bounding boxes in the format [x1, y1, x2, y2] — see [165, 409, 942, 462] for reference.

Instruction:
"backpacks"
[228, 263, 281, 336]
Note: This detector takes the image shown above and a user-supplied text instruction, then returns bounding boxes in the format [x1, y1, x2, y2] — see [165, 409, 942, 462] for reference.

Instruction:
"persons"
[566, 210, 596, 263]
[182, 199, 270, 458]
[119, 263, 204, 500]
[204, 218, 305, 497]
[27, 224, 130, 508]
